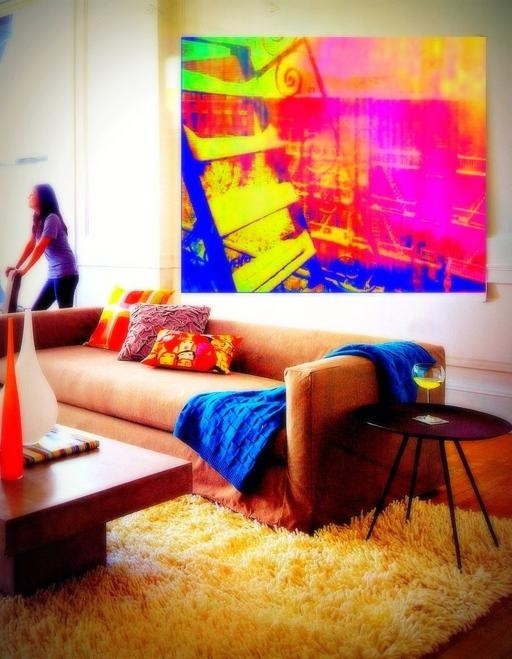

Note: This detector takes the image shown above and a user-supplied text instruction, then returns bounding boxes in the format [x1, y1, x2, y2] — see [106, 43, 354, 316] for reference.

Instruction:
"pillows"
[139, 324, 246, 375]
[80, 280, 177, 355]
[114, 300, 212, 364]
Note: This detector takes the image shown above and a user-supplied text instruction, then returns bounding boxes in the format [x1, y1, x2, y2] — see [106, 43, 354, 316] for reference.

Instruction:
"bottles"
[0, 309, 60, 448]
[1, 316, 26, 481]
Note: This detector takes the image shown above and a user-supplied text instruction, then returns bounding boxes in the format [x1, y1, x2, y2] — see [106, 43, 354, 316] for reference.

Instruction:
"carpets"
[0, 492, 512, 658]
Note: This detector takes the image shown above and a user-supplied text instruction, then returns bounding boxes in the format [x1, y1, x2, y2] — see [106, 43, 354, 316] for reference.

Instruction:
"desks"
[344, 395, 510, 572]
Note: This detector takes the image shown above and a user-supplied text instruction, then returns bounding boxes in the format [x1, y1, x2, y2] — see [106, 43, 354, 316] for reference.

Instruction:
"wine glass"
[411, 362, 451, 425]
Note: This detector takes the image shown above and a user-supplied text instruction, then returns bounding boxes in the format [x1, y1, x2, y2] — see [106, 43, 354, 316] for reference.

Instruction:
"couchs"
[1, 298, 454, 535]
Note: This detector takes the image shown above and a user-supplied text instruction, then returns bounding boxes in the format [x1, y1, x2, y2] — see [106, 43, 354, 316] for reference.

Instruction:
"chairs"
[0, 269, 22, 313]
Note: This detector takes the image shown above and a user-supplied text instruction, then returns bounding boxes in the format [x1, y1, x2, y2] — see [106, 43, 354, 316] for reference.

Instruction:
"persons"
[4, 184, 81, 313]
